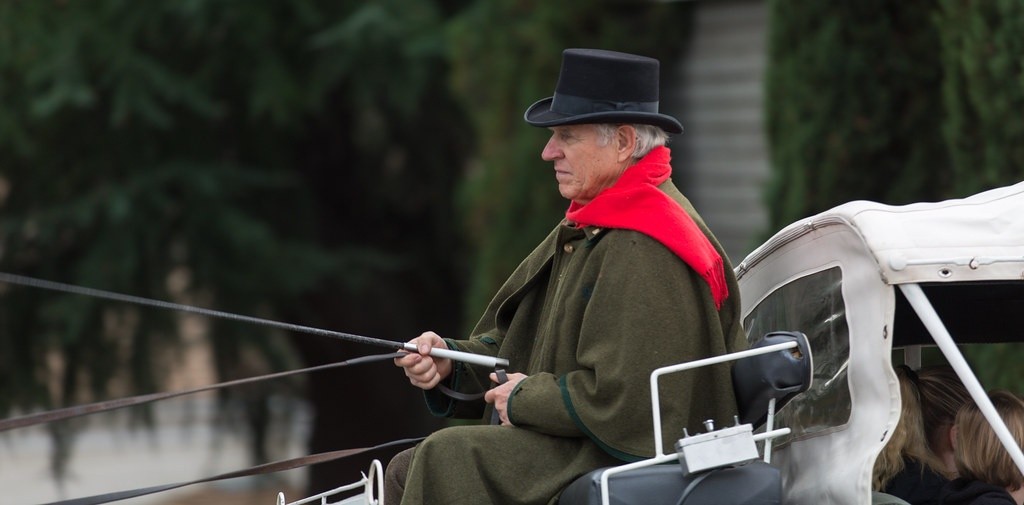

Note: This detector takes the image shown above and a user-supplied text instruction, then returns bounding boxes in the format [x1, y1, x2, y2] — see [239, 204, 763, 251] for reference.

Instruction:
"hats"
[524, 48, 685, 136]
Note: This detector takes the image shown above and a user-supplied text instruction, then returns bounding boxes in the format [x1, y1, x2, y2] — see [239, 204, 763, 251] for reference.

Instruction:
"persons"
[877, 365, 973, 504]
[376, 49, 743, 505]
[930, 389, 1024, 505]
[804, 361, 953, 505]
